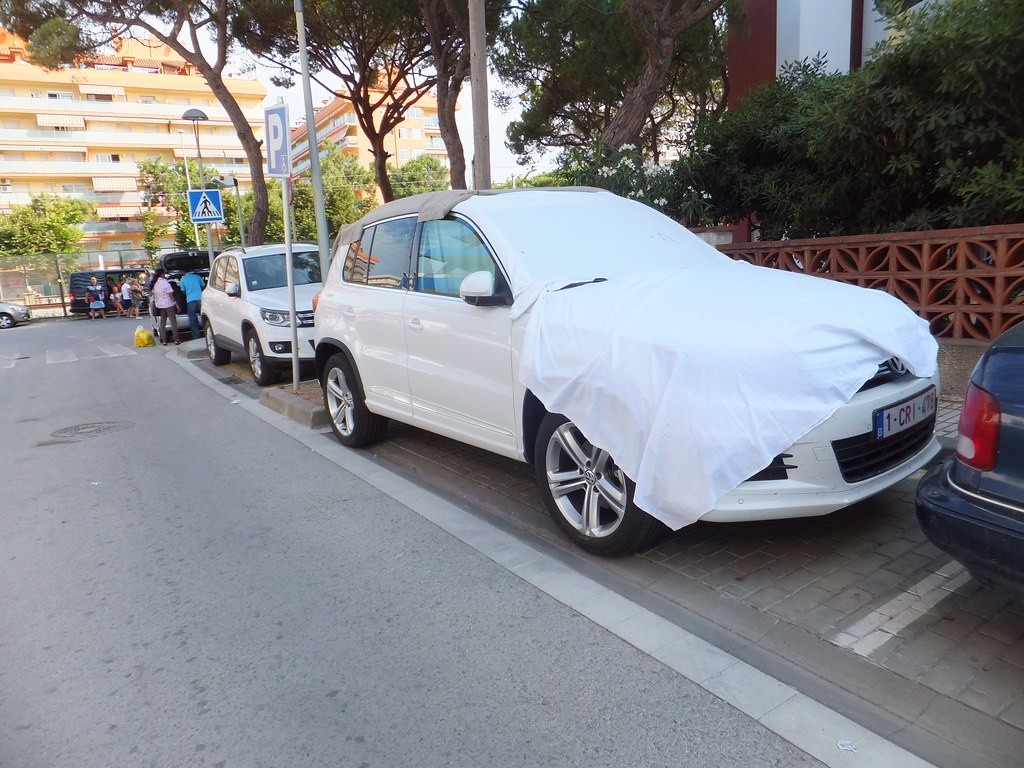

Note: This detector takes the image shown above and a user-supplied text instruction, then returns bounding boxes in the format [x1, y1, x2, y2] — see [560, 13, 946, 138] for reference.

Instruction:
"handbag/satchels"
[136, 329, 155, 347]
[89, 296, 94, 302]
[134, 326, 143, 347]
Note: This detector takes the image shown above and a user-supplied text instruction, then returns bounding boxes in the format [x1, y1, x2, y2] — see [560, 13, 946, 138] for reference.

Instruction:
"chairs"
[247, 260, 270, 291]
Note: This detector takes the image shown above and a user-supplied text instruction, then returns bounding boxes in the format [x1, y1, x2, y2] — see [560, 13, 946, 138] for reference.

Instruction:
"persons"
[109, 268, 150, 320]
[149, 269, 181, 346]
[85, 277, 107, 321]
[179, 269, 206, 339]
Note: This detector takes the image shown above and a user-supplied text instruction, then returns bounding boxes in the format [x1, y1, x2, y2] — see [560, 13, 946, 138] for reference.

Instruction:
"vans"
[69, 267, 156, 320]
[145, 248, 221, 343]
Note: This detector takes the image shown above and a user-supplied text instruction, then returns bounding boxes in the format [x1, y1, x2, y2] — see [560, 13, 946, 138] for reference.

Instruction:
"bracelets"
[173, 301, 177, 305]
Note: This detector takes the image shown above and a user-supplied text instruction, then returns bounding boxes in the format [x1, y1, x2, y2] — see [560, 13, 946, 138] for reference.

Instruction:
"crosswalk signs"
[187, 189, 225, 224]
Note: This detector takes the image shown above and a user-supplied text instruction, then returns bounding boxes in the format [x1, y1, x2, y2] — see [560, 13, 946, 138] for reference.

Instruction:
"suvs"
[313, 190, 943, 559]
[200, 241, 332, 387]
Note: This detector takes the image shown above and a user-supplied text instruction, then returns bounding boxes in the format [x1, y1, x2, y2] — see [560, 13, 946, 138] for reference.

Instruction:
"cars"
[915, 320, 1023, 589]
[0, 301, 33, 330]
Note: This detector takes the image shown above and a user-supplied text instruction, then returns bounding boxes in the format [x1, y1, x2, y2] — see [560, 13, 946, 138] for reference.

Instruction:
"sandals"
[163, 341, 167, 346]
[175, 339, 182, 345]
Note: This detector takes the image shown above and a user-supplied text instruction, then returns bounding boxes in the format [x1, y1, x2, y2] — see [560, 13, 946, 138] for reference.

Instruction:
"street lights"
[182, 109, 215, 273]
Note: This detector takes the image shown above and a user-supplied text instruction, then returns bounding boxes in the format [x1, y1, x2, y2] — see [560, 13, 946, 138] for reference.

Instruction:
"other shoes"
[130, 315, 135, 317]
[136, 317, 143, 319]
